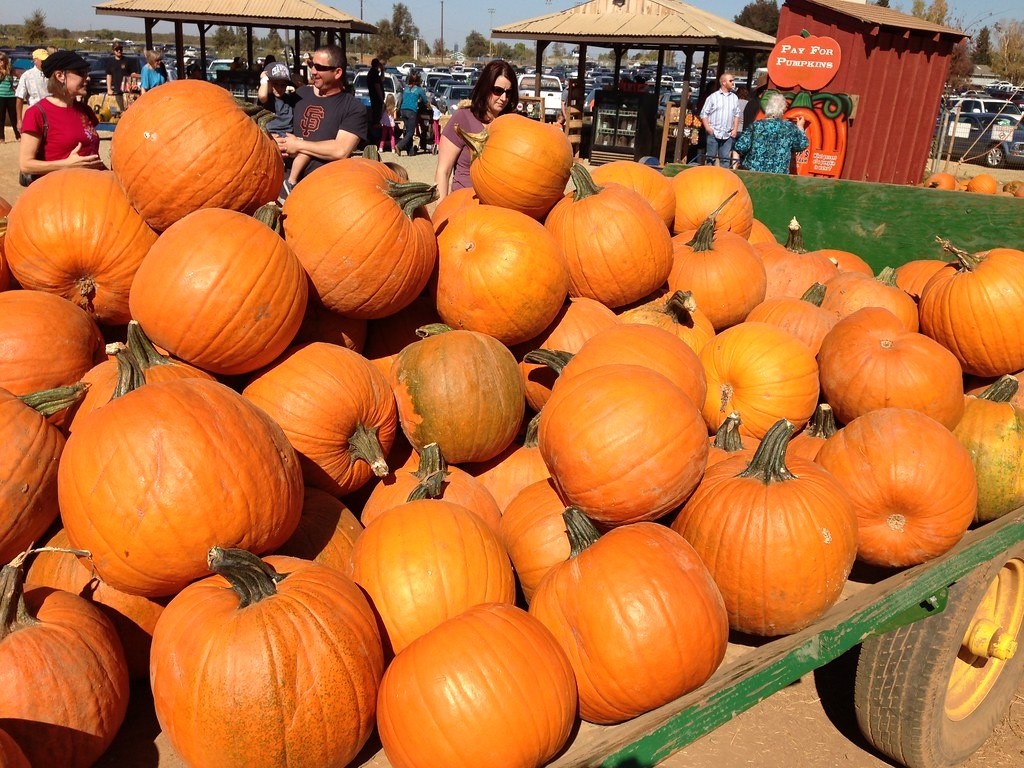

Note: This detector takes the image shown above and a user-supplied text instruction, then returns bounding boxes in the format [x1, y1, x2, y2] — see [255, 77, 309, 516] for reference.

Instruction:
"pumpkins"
[0, 145, 1024, 767]
[454, 113, 573, 219]
[110, 79, 285, 232]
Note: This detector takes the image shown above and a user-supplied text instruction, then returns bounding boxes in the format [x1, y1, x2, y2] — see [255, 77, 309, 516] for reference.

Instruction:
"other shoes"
[395, 145, 401, 156]
[392, 149, 396, 154]
[0, 138, 5, 143]
[408, 151, 415, 156]
[379, 148, 383, 152]
[17, 137, 21, 142]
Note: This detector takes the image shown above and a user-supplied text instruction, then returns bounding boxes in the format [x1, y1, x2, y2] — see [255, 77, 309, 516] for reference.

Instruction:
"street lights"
[487, 7, 496, 58]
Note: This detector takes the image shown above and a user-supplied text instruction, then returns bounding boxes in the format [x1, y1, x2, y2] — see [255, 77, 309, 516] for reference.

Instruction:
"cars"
[0, 37, 294, 99]
[930, 80, 1024, 171]
[346, 62, 767, 124]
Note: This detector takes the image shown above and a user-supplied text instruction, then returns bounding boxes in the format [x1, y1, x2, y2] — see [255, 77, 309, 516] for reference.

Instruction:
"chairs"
[963, 119, 975, 130]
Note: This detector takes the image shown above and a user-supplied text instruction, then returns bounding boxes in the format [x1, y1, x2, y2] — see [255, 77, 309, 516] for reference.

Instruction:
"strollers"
[408, 101, 442, 157]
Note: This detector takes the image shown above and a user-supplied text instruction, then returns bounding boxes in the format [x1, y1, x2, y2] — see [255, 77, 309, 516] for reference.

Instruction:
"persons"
[735, 93, 809, 175]
[105, 41, 130, 95]
[0, 51, 22, 143]
[433, 59, 519, 207]
[140, 50, 170, 96]
[556, 77, 569, 124]
[256, 45, 430, 206]
[696, 72, 770, 169]
[14, 46, 109, 181]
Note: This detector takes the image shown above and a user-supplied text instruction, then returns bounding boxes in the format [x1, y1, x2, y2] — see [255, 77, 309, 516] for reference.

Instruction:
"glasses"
[308, 61, 338, 72]
[63, 69, 90, 79]
[493, 85, 512, 99]
[115, 48, 123, 52]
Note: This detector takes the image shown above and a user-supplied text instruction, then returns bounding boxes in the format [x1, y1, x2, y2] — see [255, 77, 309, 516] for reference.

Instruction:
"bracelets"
[259, 77, 269, 84]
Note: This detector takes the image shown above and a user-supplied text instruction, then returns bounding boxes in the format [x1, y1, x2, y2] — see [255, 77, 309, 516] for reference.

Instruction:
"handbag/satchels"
[20, 103, 48, 187]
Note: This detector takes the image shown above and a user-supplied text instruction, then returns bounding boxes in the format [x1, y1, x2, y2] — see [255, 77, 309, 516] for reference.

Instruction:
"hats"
[41, 50, 90, 78]
[32, 49, 49, 61]
[112, 42, 123, 50]
[264, 62, 291, 82]
[427, 87, 434, 93]
